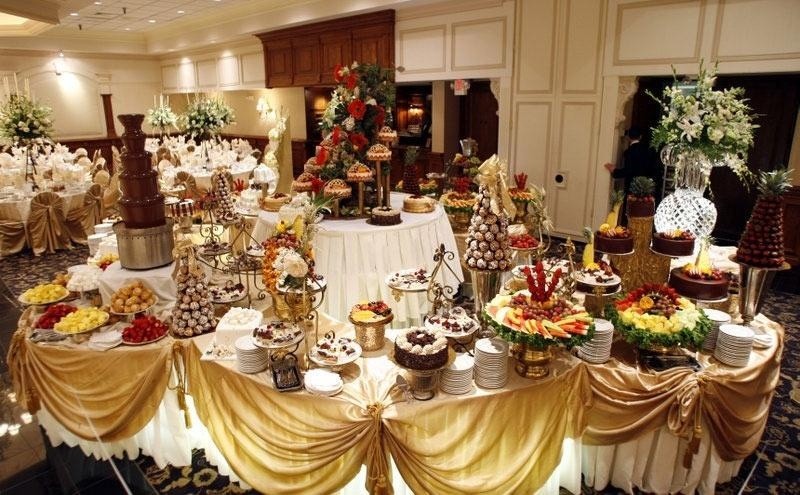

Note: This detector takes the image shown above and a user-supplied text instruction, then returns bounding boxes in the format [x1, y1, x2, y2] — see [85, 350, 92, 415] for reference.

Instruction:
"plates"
[252, 324, 305, 350]
[277, 276, 328, 294]
[714, 323, 754, 368]
[18, 289, 70, 306]
[679, 294, 729, 303]
[210, 286, 248, 303]
[474, 339, 509, 390]
[753, 332, 774, 349]
[54, 311, 109, 335]
[512, 265, 535, 275]
[354, 338, 393, 358]
[440, 352, 475, 395]
[700, 309, 732, 352]
[577, 319, 614, 364]
[403, 208, 436, 214]
[571, 270, 621, 286]
[199, 245, 232, 256]
[304, 368, 343, 398]
[110, 295, 159, 315]
[310, 340, 362, 367]
[121, 329, 170, 345]
[169, 318, 220, 339]
[366, 218, 403, 227]
[594, 248, 634, 256]
[235, 335, 270, 374]
[200, 334, 238, 363]
[583, 285, 622, 296]
[649, 247, 694, 258]
[386, 272, 432, 292]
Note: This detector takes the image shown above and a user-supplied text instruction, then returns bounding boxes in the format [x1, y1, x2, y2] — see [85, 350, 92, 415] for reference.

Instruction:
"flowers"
[637, 57, 758, 192]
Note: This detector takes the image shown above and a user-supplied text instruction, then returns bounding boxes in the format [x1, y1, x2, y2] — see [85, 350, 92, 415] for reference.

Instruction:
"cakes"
[213, 305, 264, 354]
[236, 188, 264, 213]
[570, 259, 623, 295]
[262, 192, 293, 212]
[366, 206, 402, 226]
[347, 162, 373, 182]
[593, 221, 634, 254]
[349, 300, 395, 327]
[652, 227, 697, 257]
[323, 179, 352, 197]
[303, 156, 325, 174]
[667, 234, 732, 300]
[365, 143, 392, 160]
[377, 125, 398, 142]
[290, 171, 321, 192]
[393, 323, 450, 371]
[423, 307, 481, 352]
[403, 194, 435, 213]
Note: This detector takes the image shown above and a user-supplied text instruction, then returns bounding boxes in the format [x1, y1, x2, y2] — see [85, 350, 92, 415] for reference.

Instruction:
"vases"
[652, 143, 725, 240]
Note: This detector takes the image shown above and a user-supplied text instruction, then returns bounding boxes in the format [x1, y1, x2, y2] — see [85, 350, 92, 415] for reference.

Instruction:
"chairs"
[70, 185, 104, 238]
[176, 172, 197, 201]
[0, 218, 26, 257]
[28, 192, 68, 254]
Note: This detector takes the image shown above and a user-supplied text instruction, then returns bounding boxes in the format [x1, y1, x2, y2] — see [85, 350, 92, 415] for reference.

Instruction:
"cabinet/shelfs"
[262, 39, 294, 89]
[304, 86, 432, 189]
[319, 30, 353, 86]
[351, 20, 395, 84]
[292, 34, 320, 87]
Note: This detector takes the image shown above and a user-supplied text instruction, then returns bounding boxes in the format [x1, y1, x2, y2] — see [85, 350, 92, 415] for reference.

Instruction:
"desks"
[62, 130, 268, 174]
[782, 185, 800, 267]
